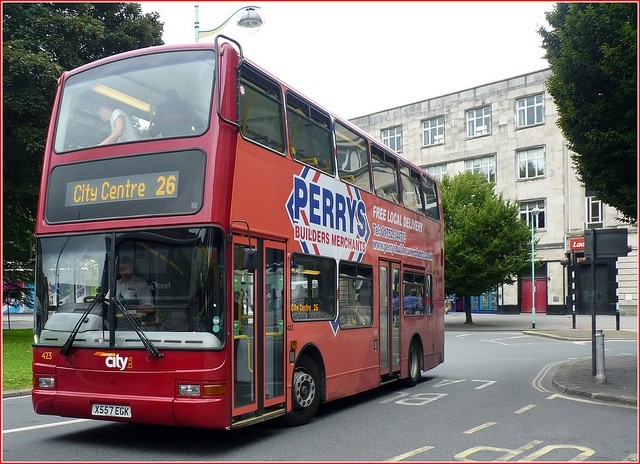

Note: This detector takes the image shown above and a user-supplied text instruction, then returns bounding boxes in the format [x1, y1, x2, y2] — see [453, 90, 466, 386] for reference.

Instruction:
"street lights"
[194, 4, 262, 43]
[531, 207, 541, 329]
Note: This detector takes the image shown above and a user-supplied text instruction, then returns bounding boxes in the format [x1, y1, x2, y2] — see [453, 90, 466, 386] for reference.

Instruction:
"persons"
[93, 100, 140, 148]
[148, 86, 206, 141]
[101, 255, 156, 320]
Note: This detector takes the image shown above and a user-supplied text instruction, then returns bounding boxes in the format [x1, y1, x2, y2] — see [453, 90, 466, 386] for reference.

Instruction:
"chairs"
[340, 174, 354, 184]
[276, 146, 296, 159]
[320, 167, 332, 174]
[300, 157, 318, 168]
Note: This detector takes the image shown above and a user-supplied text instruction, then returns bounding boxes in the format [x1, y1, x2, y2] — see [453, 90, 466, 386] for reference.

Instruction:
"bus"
[31, 34, 446, 431]
